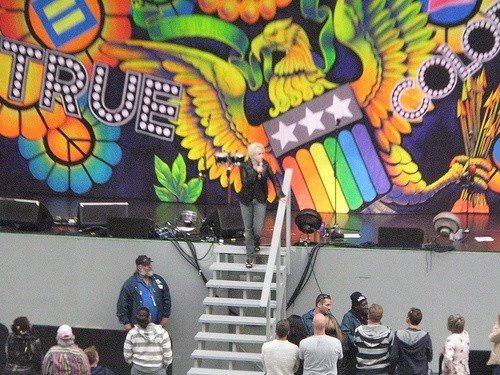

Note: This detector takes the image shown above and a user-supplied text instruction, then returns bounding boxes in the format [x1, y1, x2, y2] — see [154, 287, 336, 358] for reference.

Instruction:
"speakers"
[0, 198, 55, 232]
[360, 224, 425, 249]
[200, 208, 244, 238]
[78, 202, 156, 239]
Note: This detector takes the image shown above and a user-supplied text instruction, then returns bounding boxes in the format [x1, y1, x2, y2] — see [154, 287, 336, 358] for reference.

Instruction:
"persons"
[283, 290, 370, 375]
[116, 255, 171, 330]
[298, 313, 343, 375]
[238, 142, 288, 268]
[84, 345, 116, 375]
[41, 324, 91, 375]
[123, 307, 173, 375]
[389, 307, 432, 375]
[485, 313, 500, 375]
[353, 303, 393, 375]
[440, 312, 471, 375]
[0, 315, 42, 375]
[261, 320, 300, 375]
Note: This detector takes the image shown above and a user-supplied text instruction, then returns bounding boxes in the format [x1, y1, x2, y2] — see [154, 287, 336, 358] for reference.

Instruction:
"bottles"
[455, 228, 464, 241]
[333, 224, 341, 235]
[319, 221, 326, 238]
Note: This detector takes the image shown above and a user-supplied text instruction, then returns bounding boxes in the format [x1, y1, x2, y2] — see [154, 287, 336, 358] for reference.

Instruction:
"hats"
[349, 291, 367, 307]
[136, 255, 153, 265]
[58, 325, 73, 340]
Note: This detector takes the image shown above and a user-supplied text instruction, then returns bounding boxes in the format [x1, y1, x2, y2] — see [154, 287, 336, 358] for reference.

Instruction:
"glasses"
[318, 295, 329, 303]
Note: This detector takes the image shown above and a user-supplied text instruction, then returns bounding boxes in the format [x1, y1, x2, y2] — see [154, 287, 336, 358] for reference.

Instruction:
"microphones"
[468, 131, 473, 140]
[207, 114, 212, 122]
[335, 118, 341, 127]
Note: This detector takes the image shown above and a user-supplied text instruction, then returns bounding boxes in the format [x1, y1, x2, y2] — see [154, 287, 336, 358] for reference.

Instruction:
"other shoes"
[254, 244, 261, 252]
[246, 259, 253, 267]
